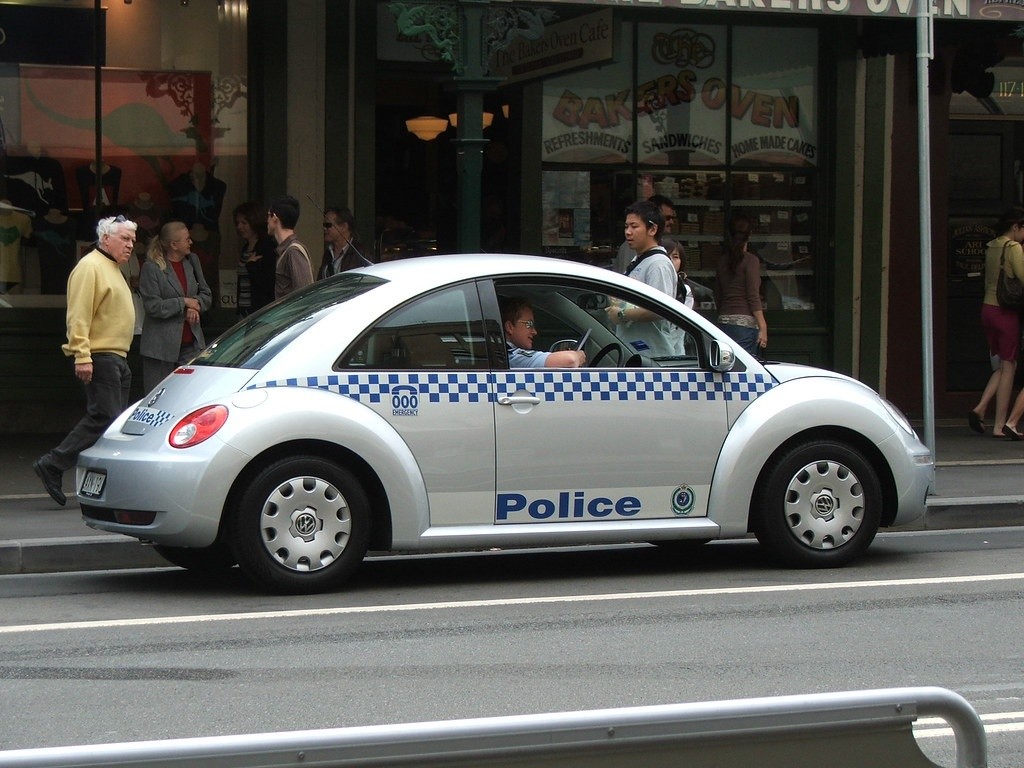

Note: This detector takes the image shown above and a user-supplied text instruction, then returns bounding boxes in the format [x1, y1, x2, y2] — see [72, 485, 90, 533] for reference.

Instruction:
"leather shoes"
[32, 457, 67, 506]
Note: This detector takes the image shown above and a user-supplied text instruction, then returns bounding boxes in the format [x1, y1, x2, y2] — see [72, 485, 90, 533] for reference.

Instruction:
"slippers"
[968, 410, 986, 434]
[1002, 425, 1024, 442]
[992, 434, 1008, 439]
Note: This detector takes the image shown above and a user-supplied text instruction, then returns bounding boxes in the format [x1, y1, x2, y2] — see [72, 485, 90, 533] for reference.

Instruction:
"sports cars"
[75, 252, 937, 595]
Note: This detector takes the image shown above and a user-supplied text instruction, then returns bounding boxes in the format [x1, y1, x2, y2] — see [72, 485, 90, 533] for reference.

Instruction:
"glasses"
[515, 320, 535, 329]
[172, 236, 191, 245]
[111, 214, 128, 223]
[322, 222, 341, 229]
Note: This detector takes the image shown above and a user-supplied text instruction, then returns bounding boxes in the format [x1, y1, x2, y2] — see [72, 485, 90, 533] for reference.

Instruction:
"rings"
[86, 374, 92, 381]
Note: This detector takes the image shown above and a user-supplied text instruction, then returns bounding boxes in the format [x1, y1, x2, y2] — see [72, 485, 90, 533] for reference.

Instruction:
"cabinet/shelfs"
[615, 169, 815, 311]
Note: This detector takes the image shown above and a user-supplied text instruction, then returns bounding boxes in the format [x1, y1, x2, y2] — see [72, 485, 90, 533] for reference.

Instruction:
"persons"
[317, 207, 369, 283]
[0, 198, 80, 295]
[604, 193, 695, 359]
[74, 157, 122, 209]
[499, 294, 586, 369]
[33, 214, 138, 507]
[968, 208, 1024, 442]
[716, 212, 768, 357]
[168, 163, 227, 308]
[232, 195, 315, 326]
[139, 222, 213, 398]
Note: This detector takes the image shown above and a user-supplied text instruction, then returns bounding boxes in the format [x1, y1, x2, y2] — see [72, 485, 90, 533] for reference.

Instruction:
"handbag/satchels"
[996, 239, 1024, 311]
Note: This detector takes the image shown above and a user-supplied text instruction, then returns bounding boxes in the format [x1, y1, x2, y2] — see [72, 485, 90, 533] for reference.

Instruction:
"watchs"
[617, 308, 628, 324]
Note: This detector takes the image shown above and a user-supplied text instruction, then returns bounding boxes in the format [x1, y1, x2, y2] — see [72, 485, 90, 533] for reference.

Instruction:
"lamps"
[405, 80, 493, 141]
